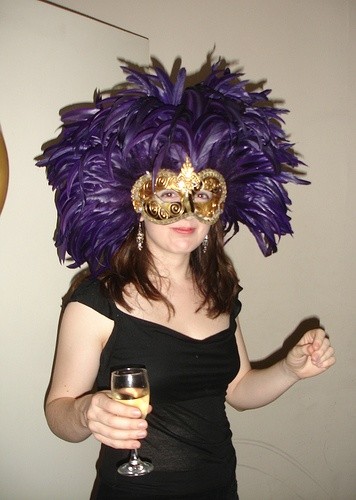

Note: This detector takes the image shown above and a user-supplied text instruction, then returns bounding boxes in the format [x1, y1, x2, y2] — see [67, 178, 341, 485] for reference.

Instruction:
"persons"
[35, 57, 337, 499]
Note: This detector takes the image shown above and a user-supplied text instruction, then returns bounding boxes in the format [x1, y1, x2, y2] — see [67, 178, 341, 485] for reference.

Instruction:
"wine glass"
[111, 367, 155, 476]
